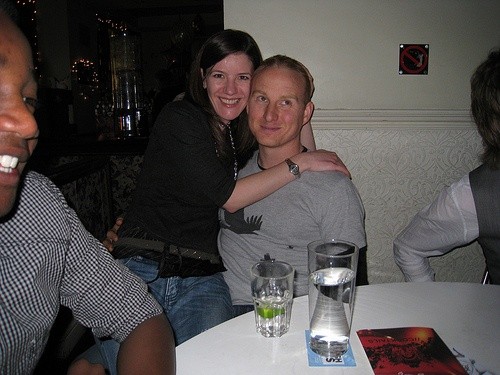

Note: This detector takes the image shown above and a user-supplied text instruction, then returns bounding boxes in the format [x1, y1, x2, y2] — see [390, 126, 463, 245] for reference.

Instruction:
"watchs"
[286, 158, 301, 179]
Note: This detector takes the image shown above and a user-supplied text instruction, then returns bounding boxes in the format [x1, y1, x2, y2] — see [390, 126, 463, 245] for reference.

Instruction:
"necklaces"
[214, 121, 238, 181]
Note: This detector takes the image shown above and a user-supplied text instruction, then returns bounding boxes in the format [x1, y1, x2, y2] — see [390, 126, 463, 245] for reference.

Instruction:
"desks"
[173, 281, 500, 375]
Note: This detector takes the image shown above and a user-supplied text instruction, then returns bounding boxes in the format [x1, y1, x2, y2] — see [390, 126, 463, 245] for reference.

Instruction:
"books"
[357, 327, 471, 375]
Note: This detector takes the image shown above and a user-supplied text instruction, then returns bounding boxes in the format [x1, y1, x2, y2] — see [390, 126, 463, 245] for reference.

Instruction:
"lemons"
[256, 306, 284, 318]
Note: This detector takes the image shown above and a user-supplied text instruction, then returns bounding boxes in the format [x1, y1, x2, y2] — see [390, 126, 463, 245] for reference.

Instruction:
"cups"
[250, 261, 295, 337]
[307, 239, 359, 359]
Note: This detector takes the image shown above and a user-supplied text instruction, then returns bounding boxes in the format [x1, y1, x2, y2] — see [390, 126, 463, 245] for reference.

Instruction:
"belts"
[112, 237, 220, 264]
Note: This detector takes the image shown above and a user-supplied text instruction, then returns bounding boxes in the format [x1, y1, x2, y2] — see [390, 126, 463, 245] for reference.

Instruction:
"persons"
[0, 7, 176, 375]
[67, 55, 366, 375]
[393, 47, 500, 286]
[91, 28, 353, 375]
